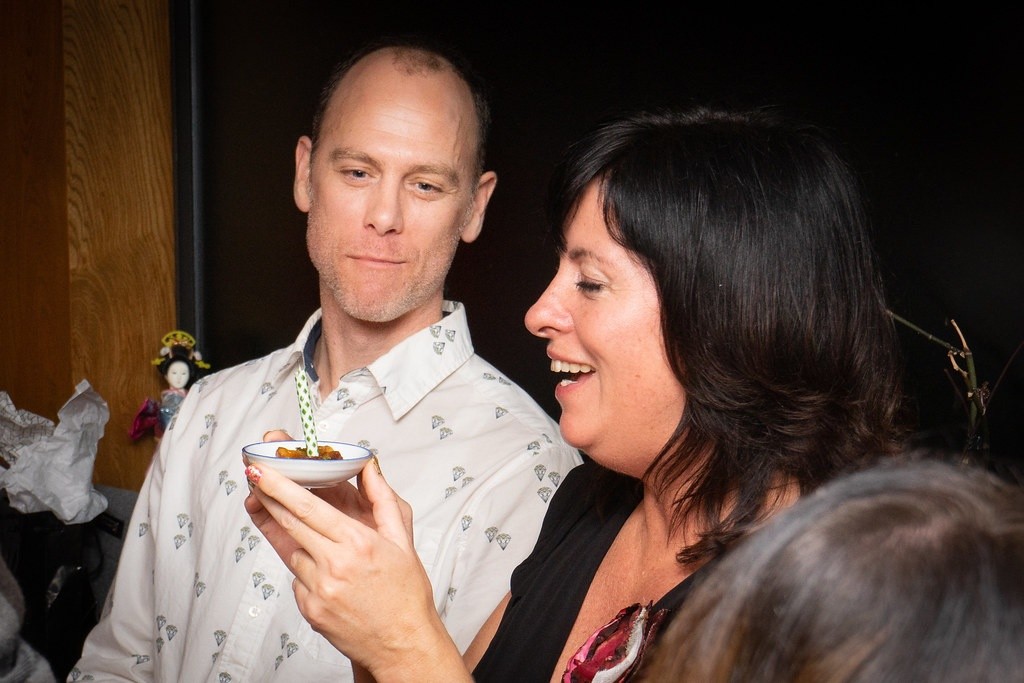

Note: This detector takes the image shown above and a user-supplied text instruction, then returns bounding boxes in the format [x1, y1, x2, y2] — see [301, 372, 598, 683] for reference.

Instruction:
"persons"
[246, 107, 912, 683]
[61, 45, 577, 683]
[555, 442, 1023, 681]
[124, 333, 211, 449]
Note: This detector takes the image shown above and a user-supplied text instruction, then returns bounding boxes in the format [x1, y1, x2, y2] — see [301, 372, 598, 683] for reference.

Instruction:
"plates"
[240, 439, 372, 487]
